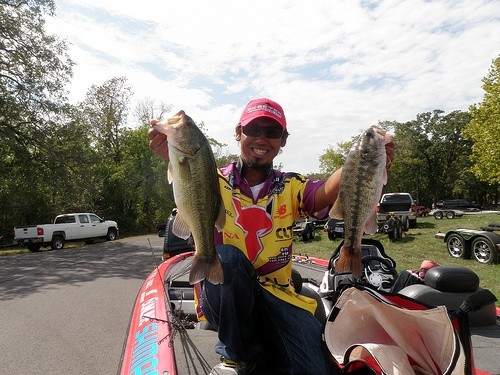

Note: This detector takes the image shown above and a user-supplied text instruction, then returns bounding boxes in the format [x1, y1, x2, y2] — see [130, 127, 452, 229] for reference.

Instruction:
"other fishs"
[151, 108, 227, 287]
[328, 122, 393, 280]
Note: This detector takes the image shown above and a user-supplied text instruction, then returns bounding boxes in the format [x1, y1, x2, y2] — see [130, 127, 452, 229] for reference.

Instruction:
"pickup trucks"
[13, 212, 120, 252]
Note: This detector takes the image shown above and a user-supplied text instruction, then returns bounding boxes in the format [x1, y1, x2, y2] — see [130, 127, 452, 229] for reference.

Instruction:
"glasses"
[242, 122, 285, 139]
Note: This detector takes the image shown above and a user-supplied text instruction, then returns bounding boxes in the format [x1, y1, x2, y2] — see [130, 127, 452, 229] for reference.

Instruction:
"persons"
[148, 98, 394, 375]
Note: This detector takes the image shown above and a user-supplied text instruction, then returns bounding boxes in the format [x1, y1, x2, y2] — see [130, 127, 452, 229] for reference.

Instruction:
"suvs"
[433, 198, 481, 218]
[375, 192, 418, 229]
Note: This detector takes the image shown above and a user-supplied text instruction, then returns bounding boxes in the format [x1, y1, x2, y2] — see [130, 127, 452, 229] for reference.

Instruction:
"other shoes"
[208, 352, 256, 375]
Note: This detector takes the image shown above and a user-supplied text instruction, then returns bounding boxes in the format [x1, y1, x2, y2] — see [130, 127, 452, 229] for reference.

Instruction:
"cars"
[323, 218, 345, 241]
[292, 215, 316, 241]
[158, 207, 197, 262]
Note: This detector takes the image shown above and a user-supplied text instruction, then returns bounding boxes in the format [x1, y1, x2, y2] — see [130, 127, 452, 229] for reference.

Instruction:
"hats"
[239, 98, 288, 133]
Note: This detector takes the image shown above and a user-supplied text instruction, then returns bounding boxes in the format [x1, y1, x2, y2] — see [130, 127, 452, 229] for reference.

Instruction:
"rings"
[386, 158, 391, 166]
